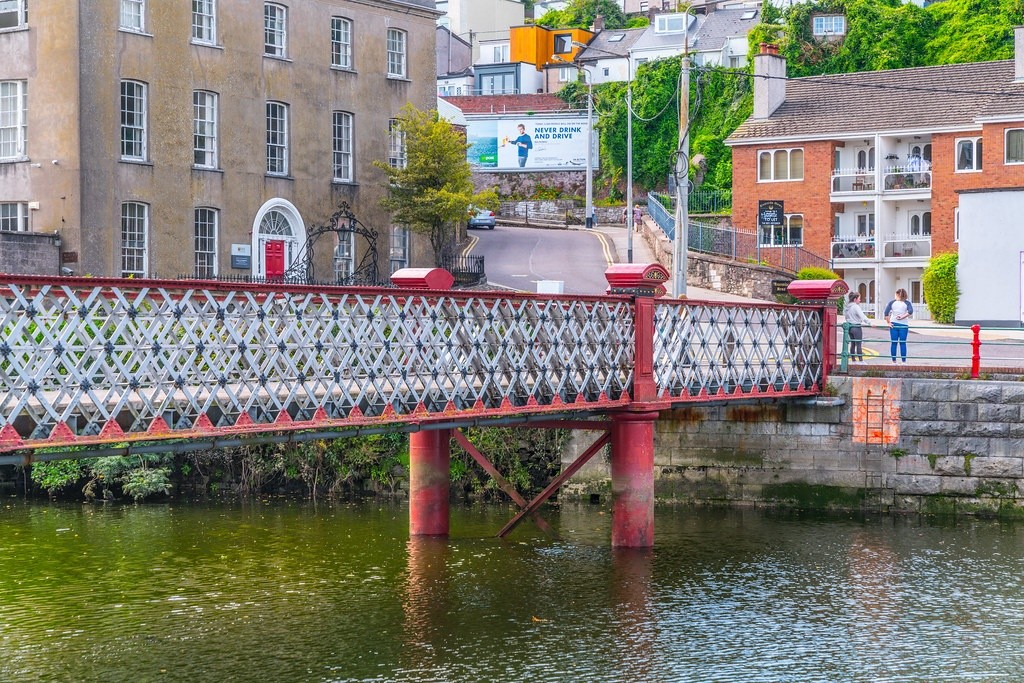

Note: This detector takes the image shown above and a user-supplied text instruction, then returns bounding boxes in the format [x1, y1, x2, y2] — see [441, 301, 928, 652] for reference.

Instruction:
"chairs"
[853, 173, 865, 191]
[901, 241, 916, 256]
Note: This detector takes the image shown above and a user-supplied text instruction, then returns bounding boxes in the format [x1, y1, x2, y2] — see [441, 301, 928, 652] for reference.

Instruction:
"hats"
[592, 201, 594, 204]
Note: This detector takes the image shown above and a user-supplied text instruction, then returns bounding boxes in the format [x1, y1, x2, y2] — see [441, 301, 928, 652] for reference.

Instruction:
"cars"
[467, 201, 496, 229]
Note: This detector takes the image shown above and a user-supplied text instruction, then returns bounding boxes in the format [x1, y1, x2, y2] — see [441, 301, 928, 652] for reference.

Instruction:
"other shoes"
[596, 224, 600, 227]
[901, 363, 908, 366]
[851, 360, 858, 364]
[858, 361, 868, 366]
[892, 362, 898, 367]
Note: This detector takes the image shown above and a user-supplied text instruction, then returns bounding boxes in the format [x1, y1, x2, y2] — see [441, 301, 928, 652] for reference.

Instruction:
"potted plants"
[891, 169, 901, 189]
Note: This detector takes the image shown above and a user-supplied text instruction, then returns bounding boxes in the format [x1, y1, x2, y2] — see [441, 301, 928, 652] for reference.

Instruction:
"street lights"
[572, 41, 632, 263]
[553, 55, 592, 228]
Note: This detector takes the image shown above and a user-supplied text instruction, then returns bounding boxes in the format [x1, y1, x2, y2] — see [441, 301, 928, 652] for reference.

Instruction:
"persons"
[846, 292, 872, 361]
[507, 124, 533, 167]
[883, 289, 913, 363]
[591, 202, 600, 227]
[624, 204, 644, 233]
